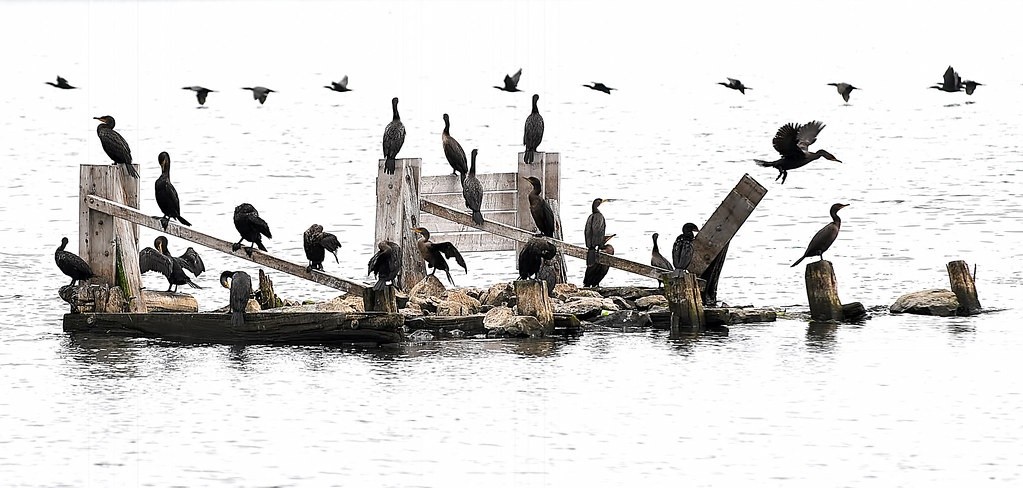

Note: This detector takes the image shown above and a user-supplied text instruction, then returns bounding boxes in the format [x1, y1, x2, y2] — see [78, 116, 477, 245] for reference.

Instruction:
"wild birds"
[826, 82, 863, 102]
[753, 120, 843, 185]
[929, 65, 987, 96]
[582, 81, 619, 95]
[790, 203, 850, 268]
[492, 67, 526, 93]
[323, 74, 354, 92]
[715, 77, 754, 96]
[44, 75, 78, 89]
[180, 85, 220, 106]
[240, 86, 279, 106]
[54, 94, 702, 327]
